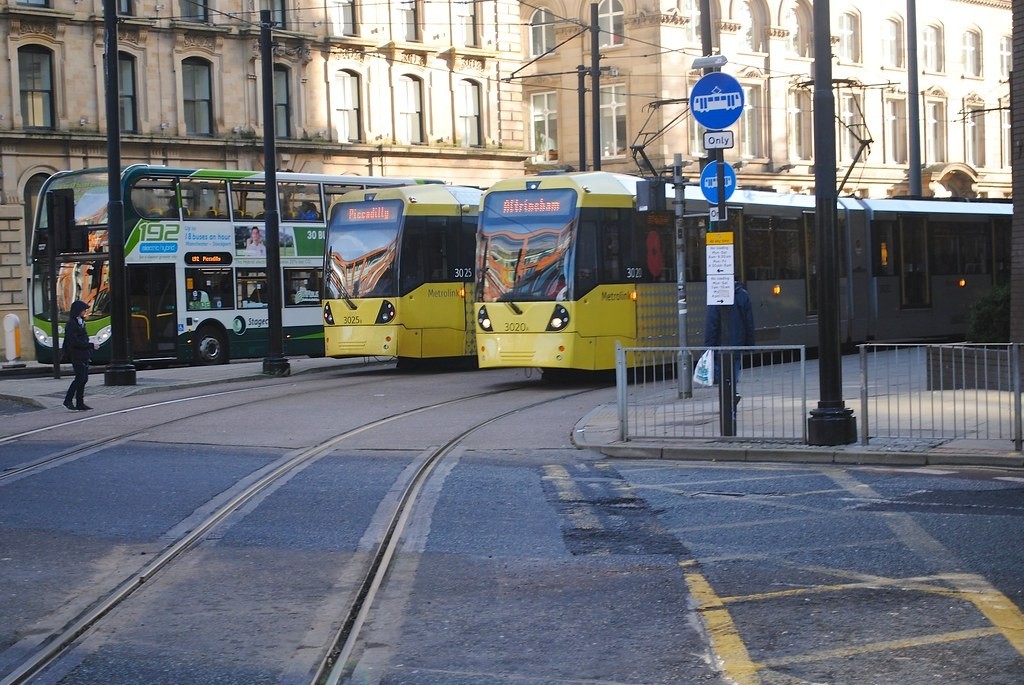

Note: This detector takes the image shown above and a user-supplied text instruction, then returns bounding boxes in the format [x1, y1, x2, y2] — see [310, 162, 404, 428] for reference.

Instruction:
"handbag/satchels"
[693, 350, 714, 387]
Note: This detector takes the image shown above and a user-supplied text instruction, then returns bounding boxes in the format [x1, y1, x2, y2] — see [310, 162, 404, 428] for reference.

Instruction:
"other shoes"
[62, 401, 76, 412]
[76, 404, 94, 412]
[736, 392, 741, 404]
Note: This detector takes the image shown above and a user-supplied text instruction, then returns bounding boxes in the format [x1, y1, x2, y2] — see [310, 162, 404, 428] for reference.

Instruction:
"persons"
[246, 226, 266, 256]
[62, 301, 100, 412]
[294, 202, 317, 221]
[706, 280, 754, 405]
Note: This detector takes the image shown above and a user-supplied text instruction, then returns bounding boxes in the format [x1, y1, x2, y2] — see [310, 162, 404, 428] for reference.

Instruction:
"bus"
[25, 165, 450, 365]
[473, 80, 1015, 381]
[322, 186, 480, 361]
